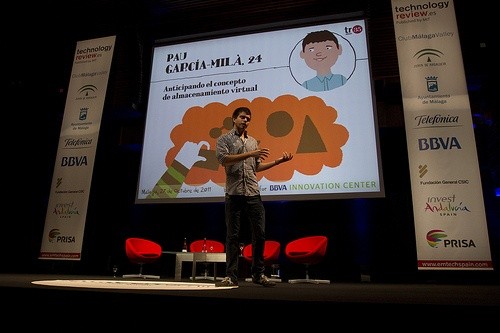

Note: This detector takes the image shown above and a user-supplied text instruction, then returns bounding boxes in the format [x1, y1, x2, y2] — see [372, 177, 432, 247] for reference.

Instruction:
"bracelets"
[275, 160, 279, 165]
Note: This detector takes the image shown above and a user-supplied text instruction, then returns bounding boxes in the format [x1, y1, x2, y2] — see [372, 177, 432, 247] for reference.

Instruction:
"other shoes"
[252, 272, 276, 287]
[215, 276, 238, 287]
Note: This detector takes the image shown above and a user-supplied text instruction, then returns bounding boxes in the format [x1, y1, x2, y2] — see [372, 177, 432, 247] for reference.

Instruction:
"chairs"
[243, 240, 282, 282]
[122, 237, 162, 281]
[190, 239, 226, 282]
[285, 236, 331, 284]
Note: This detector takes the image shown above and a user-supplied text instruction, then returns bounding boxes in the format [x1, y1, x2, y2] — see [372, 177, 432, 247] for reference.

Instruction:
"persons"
[215, 107, 293, 287]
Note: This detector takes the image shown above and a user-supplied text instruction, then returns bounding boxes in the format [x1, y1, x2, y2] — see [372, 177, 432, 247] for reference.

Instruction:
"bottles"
[183, 238, 188, 253]
[113, 264, 118, 278]
[202, 238, 208, 252]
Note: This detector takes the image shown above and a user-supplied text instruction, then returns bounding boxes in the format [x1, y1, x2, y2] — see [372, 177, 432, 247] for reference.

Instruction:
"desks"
[175, 252, 228, 283]
